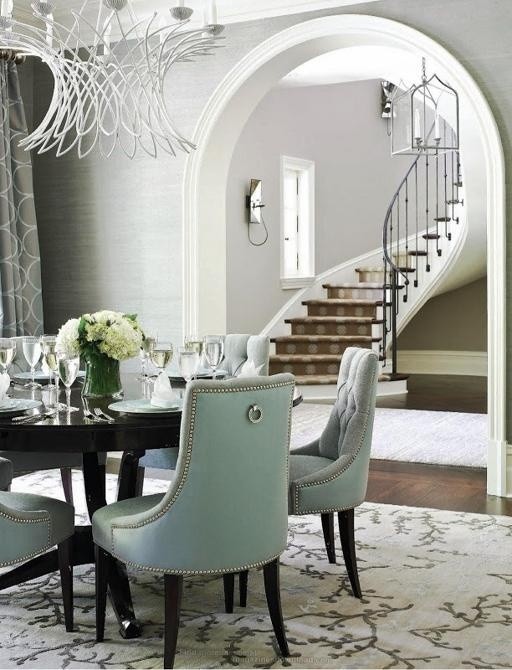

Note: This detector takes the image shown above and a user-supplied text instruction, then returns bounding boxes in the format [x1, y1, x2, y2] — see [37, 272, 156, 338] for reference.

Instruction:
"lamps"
[389, 56, 460, 158]
[245, 179, 268, 246]
[0, 0, 224, 160]
[380, 80, 398, 136]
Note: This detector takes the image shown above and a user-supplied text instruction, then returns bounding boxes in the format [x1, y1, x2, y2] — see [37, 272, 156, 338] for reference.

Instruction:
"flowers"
[51, 303, 142, 362]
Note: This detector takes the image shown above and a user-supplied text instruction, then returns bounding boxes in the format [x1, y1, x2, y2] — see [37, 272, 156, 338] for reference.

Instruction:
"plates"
[108, 398, 184, 418]
[1, 398, 43, 414]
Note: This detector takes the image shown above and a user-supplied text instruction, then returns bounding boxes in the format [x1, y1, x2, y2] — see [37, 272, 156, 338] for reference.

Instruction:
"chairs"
[0, 333, 379, 670]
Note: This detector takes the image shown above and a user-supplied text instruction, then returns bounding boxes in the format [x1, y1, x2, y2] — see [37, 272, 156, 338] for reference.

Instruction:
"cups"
[150, 340, 175, 373]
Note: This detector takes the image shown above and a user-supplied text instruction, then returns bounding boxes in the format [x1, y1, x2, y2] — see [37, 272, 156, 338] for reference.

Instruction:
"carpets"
[0, 469, 512, 670]
[290, 396, 487, 470]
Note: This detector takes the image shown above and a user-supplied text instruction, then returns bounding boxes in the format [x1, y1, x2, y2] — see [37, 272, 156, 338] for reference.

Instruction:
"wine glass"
[180, 336, 224, 383]
[136, 329, 160, 385]
[57, 351, 80, 412]
[0, 337, 18, 410]
[24, 333, 60, 393]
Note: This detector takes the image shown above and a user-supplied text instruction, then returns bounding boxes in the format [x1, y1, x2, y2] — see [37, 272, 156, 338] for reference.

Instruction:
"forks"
[83, 407, 115, 423]
[25, 407, 60, 424]
